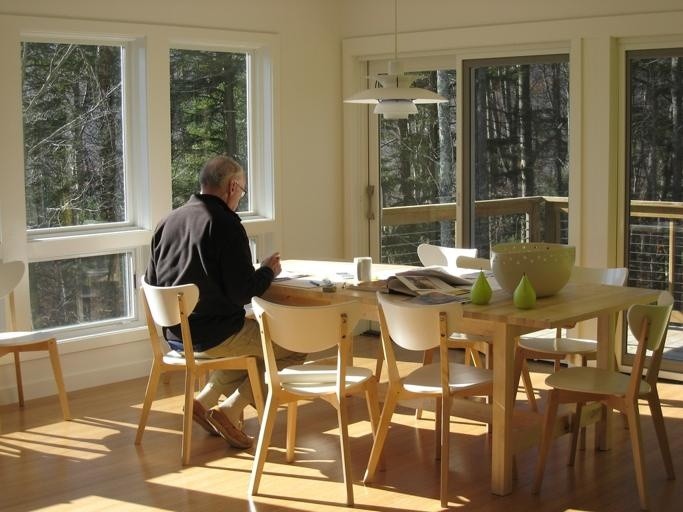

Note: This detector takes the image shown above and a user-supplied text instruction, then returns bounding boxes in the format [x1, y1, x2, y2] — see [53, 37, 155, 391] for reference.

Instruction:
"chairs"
[249, 296, 386, 506]
[362, 291, 493, 507]
[134, 275, 265, 467]
[0, 260, 72, 422]
[531, 290, 676, 510]
[512, 268, 627, 451]
[416, 243, 494, 434]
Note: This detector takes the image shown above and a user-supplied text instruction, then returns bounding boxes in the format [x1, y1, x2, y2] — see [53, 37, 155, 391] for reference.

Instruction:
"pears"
[471, 272, 493, 305]
[513, 275, 537, 310]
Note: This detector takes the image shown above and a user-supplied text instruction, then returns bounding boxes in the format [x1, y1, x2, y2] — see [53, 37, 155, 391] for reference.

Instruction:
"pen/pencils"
[310, 280, 319, 286]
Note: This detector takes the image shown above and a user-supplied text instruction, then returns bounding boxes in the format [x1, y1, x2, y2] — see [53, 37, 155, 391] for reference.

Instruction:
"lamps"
[342, 0, 450, 119]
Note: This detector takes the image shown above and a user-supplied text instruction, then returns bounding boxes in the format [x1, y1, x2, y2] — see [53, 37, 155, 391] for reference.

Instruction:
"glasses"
[235, 183, 247, 198]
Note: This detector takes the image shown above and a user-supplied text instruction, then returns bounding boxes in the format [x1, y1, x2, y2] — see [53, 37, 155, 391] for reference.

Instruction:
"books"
[347, 267, 475, 304]
[273, 274, 336, 290]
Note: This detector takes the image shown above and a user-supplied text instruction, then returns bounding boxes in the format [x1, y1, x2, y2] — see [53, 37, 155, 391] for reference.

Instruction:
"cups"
[354, 257, 372, 284]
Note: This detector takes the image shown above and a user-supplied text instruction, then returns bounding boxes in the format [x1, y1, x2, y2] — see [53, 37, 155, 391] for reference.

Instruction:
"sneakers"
[206, 405, 255, 449]
[183, 399, 220, 437]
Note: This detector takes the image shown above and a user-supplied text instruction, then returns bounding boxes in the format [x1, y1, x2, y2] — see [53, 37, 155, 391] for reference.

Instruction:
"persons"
[146, 155, 282, 448]
